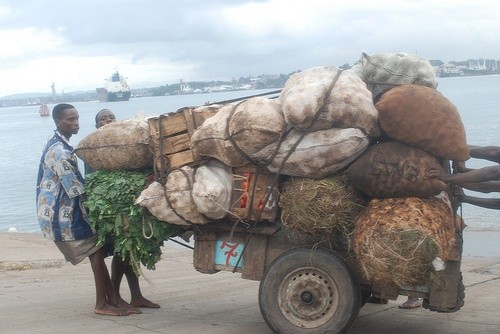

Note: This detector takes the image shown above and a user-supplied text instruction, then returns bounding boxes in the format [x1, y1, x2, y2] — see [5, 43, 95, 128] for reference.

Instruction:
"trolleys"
[87, 52, 468, 334]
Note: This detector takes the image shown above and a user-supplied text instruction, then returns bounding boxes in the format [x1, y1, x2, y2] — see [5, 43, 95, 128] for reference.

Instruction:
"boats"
[39, 104, 49, 117]
[106, 72, 131, 101]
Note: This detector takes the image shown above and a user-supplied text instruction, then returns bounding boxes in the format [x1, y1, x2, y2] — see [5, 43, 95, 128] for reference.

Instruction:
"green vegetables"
[83, 170, 184, 279]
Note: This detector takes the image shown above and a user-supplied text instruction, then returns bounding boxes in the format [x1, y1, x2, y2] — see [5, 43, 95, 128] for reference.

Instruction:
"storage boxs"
[226, 161, 284, 223]
[148, 103, 222, 173]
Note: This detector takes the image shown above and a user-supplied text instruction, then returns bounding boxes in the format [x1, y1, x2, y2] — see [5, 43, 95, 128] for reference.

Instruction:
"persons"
[426, 142, 499, 211]
[82, 107, 161, 310]
[35, 103, 143, 317]
